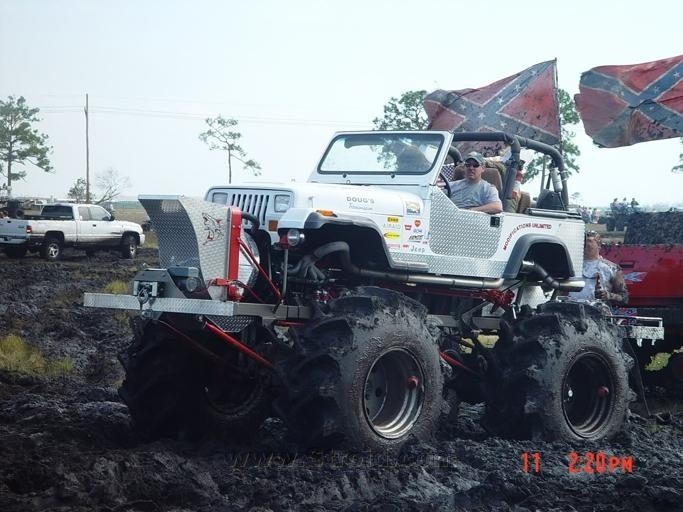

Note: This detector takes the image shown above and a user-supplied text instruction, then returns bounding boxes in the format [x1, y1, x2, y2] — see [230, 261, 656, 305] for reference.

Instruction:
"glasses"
[465, 164, 479, 167]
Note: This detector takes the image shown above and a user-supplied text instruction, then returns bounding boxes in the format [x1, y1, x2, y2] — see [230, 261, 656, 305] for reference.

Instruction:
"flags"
[577, 55, 682, 146]
[424, 58, 560, 156]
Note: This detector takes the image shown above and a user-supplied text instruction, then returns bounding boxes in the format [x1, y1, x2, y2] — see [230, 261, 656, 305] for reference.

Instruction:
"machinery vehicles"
[78, 196, 683, 453]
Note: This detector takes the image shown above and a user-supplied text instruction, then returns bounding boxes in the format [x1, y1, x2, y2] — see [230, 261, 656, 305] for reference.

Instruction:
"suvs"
[604, 206, 647, 232]
[204, 128, 587, 306]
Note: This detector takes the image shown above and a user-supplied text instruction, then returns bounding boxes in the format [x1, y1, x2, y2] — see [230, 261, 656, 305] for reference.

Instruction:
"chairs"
[452, 163, 521, 212]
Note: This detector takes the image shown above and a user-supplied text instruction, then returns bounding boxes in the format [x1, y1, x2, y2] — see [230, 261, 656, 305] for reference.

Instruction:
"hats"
[464, 152, 484, 165]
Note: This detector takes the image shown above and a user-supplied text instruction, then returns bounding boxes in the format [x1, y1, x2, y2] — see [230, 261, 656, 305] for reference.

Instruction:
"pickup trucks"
[0, 201, 145, 261]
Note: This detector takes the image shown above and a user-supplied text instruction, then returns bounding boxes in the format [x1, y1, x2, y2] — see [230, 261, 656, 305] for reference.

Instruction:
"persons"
[434, 151, 502, 215]
[559, 230, 632, 306]
